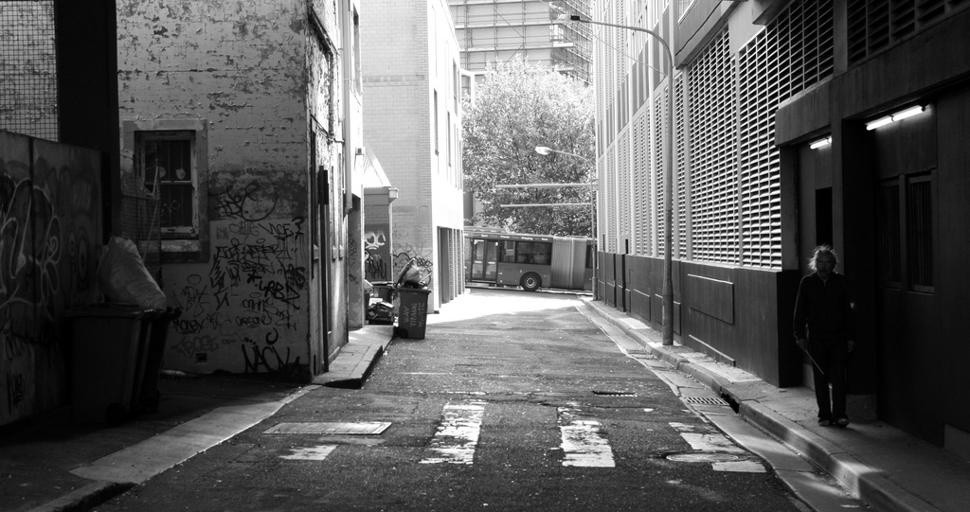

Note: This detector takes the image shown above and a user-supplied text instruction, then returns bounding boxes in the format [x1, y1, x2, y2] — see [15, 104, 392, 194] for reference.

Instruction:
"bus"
[459, 226, 598, 293]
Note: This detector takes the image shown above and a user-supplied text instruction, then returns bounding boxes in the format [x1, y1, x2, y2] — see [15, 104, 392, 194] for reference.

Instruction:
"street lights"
[557, 13, 676, 347]
[534, 145, 603, 304]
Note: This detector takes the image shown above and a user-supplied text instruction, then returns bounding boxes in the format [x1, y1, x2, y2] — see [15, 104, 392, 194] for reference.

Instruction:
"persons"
[792, 246, 858, 429]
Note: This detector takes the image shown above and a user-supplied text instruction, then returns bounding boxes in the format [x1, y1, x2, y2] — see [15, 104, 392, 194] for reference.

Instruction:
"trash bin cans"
[395, 288, 431, 339]
[65, 304, 182, 426]
[371, 281, 394, 305]
